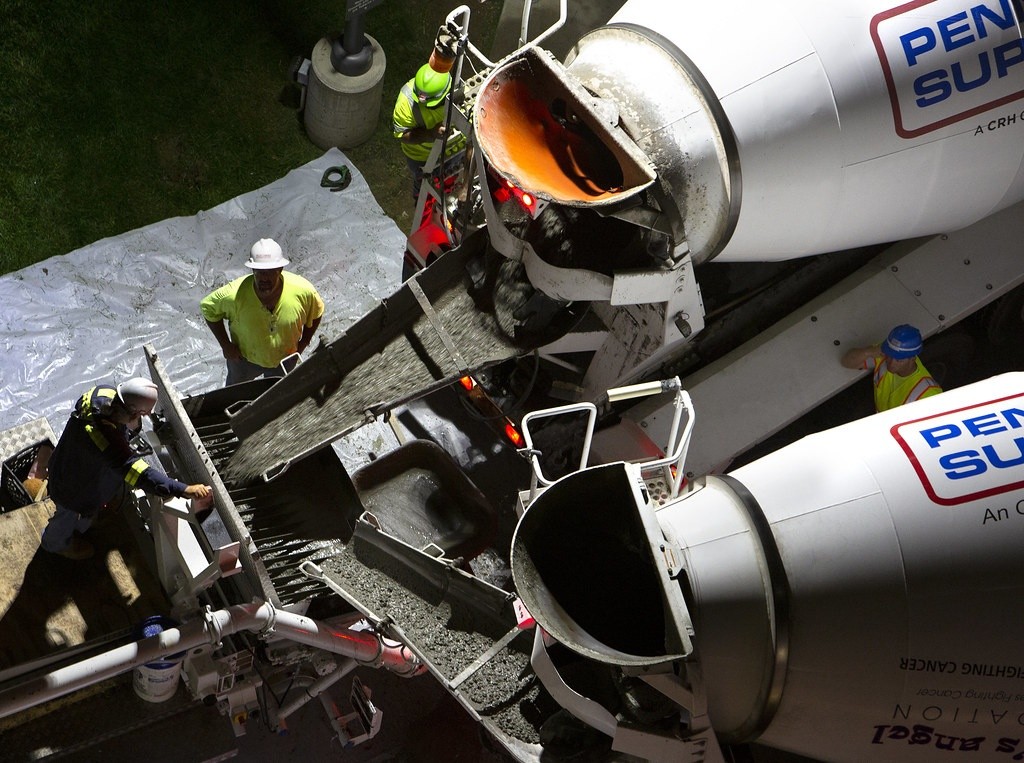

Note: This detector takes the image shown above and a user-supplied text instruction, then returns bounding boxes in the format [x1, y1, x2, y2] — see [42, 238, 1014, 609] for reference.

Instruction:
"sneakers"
[42, 532, 95, 560]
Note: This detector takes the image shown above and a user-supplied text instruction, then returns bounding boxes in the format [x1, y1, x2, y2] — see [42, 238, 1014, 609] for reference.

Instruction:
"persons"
[841, 324, 943, 413]
[393, 63, 480, 208]
[38, 377, 210, 563]
[201, 238, 325, 388]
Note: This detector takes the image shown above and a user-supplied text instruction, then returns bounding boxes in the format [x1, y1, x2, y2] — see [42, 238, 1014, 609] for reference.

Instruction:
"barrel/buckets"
[133, 615, 186, 702]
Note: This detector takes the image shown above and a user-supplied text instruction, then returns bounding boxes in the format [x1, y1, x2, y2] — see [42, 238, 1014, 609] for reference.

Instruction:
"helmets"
[412, 63, 452, 107]
[117, 378, 158, 414]
[245, 238, 289, 269]
[881, 324, 923, 359]
